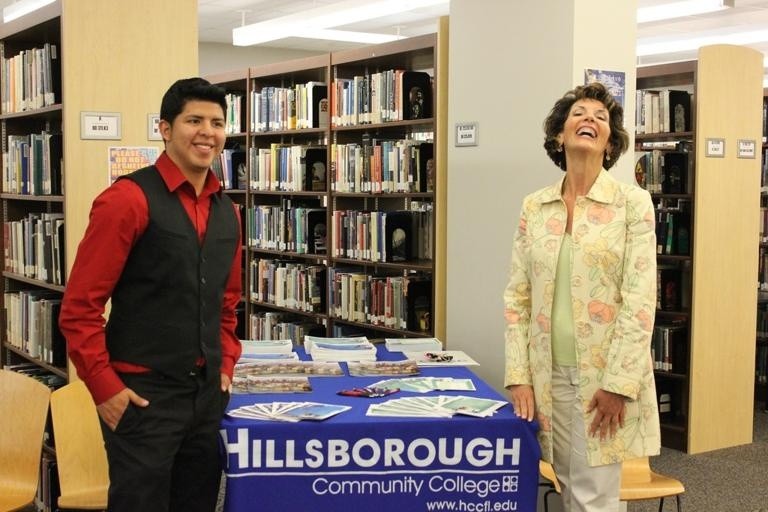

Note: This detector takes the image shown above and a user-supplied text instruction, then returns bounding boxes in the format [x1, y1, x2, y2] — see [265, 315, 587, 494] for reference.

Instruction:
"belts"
[190, 366, 206, 375]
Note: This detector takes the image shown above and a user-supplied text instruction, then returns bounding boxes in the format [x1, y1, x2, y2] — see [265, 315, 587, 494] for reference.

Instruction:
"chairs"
[50, 378, 109, 510]
[0, 368, 52, 512]
[539, 456, 686, 510]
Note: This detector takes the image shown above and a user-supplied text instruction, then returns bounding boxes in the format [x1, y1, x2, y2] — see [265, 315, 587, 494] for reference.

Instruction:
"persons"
[61, 78, 241, 512]
[505, 83, 660, 512]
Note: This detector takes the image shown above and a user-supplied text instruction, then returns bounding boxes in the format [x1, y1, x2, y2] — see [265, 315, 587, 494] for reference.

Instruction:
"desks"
[226, 329, 541, 509]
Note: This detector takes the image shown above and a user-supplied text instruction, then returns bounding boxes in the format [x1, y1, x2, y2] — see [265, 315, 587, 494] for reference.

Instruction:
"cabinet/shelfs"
[206, 71, 250, 347]
[0, 12, 67, 357]
[247, 51, 332, 342]
[635, 63, 694, 457]
[331, 53, 440, 341]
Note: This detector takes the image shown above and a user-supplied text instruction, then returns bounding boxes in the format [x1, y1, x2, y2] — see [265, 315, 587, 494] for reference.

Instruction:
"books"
[250, 80, 327, 343]
[330, 69, 434, 339]
[635, 88, 693, 417]
[3, 41, 60, 385]
[36, 447, 59, 509]
[210, 94, 247, 191]
[304, 336, 443, 361]
[237, 339, 299, 364]
